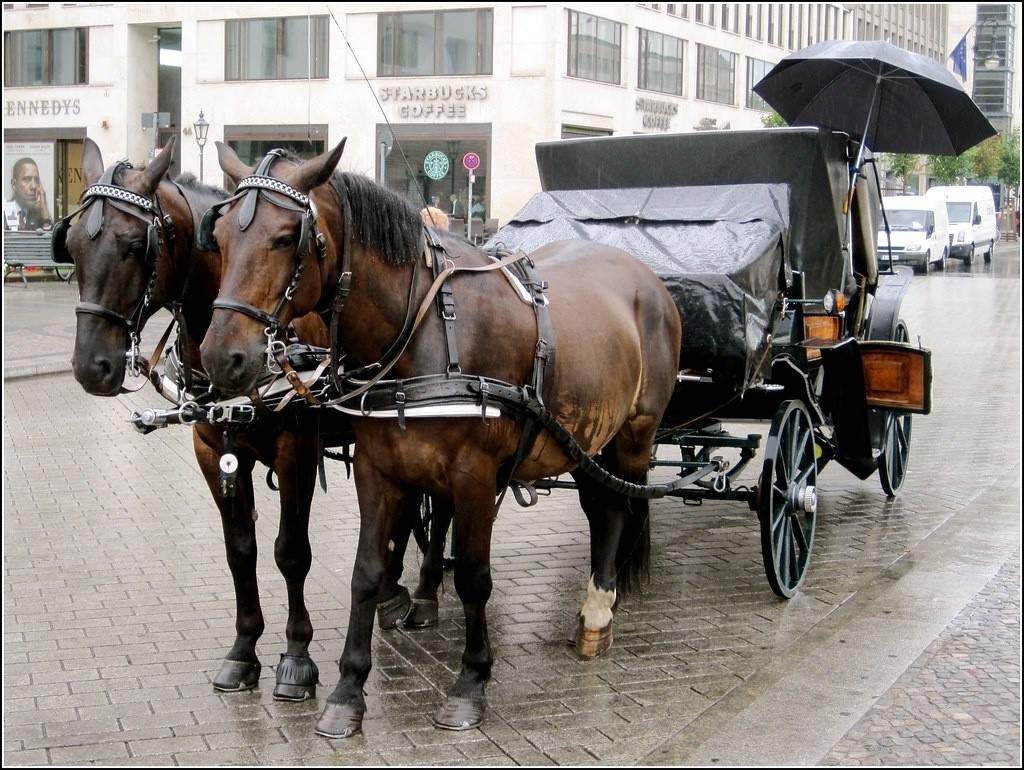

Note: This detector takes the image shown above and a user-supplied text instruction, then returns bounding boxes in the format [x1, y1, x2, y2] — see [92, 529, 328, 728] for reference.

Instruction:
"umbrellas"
[750, 37, 999, 214]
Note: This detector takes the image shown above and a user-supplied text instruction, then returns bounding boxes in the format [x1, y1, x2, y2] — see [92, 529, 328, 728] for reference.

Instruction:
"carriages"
[52, 124, 932, 740]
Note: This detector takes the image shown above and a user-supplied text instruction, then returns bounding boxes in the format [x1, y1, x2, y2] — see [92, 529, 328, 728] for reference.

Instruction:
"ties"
[18, 211, 26, 230]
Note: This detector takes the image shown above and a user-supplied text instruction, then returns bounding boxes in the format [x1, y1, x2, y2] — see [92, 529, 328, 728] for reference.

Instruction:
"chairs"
[448, 219, 468, 238]
[485, 218, 499, 243]
[470, 218, 489, 247]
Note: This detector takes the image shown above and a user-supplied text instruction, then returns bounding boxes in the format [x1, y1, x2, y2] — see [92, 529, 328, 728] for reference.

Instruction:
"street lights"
[192, 109, 210, 186]
[970, 18, 1002, 103]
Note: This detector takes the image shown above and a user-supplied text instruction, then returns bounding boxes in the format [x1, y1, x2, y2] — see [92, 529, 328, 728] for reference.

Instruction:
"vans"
[876, 196, 949, 276]
[926, 185, 997, 267]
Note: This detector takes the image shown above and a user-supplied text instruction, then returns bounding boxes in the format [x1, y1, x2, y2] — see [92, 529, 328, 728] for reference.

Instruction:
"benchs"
[4, 230, 76, 289]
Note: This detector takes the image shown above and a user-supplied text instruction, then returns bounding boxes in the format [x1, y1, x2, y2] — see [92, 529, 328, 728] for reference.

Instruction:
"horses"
[63, 136, 454, 702]
[198, 134, 683, 739]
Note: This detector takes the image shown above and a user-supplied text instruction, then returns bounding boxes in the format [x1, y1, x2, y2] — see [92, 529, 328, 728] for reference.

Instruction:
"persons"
[3, 158, 54, 232]
[450, 193, 465, 219]
[428, 194, 442, 209]
